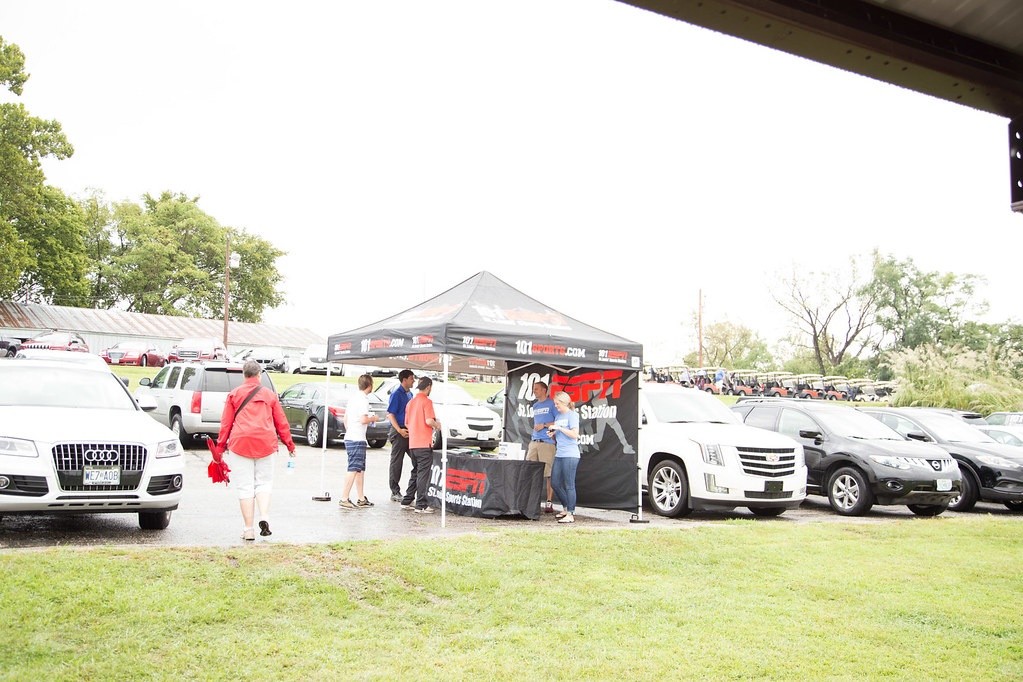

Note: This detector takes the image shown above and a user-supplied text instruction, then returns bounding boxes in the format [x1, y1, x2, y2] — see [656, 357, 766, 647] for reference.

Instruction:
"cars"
[229, 344, 345, 376]
[98, 339, 168, 367]
[167, 338, 228, 364]
[480, 385, 504, 414]
[365, 365, 397, 377]
[0, 348, 186, 529]
[278, 381, 392, 448]
[851, 407, 1023, 512]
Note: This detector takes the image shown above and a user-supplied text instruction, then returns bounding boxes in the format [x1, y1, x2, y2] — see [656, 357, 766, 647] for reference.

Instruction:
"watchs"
[543, 423, 547, 430]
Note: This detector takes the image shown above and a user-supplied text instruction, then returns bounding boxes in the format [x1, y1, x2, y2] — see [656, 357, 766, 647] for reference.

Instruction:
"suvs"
[374, 377, 501, 451]
[133, 359, 280, 449]
[729, 394, 962, 516]
[639, 383, 808, 516]
[18, 331, 90, 351]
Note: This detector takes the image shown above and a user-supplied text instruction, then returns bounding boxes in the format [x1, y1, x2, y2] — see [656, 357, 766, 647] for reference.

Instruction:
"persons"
[339, 374, 379, 510]
[217, 361, 295, 540]
[526, 381, 560, 513]
[387, 370, 416, 504]
[546, 391, 581, 523]
[715, 366, 725, 395]
[398, 378, 441, 513]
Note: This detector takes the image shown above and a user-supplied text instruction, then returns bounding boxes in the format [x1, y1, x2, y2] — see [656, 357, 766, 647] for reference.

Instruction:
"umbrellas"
[206, 435, 231, 483]
[312, 271, 649, 528]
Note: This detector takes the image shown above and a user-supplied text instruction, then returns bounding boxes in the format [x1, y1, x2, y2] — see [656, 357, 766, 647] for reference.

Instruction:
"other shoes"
[415, 506, 435, 513]
[558, 512, 575, 522]
[357, 496, 374, 507]
[240, 527, 255, 540]
[556, 511, 567, 517]
[391, 494, 404, 502]
[545, 501, 553, 513]
[339, 498, 361, 510]
[401, 501, 416, 509]
[259, 520, 272, 536]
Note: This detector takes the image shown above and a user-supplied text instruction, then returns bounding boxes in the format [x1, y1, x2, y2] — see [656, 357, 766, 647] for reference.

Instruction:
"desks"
[428, 449, 546, 520]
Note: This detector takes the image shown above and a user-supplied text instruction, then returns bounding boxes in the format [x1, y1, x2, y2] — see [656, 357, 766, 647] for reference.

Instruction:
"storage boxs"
[498, 442, 527, 460]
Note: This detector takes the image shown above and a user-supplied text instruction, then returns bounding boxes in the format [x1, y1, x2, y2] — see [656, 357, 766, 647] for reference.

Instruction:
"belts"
[534, 440, 544, 442]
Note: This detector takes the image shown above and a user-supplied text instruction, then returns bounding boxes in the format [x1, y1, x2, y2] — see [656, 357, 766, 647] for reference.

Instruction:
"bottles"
[286, 454, 295, 477]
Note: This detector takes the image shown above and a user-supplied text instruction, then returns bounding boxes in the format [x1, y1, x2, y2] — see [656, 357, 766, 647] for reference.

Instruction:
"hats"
[415, 376, 432, 389]
